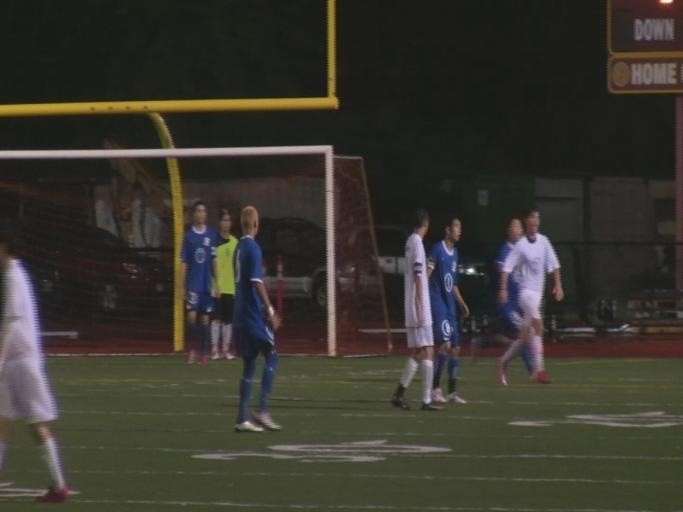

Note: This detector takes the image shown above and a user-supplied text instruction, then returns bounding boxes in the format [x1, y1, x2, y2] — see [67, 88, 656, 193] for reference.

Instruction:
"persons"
[597, 297, 613, 320]
[388, 204, 444, 413]
[230, 204, 284, 435]
[492, 209, 567, 389]
[208, 205, 241, 362]
[484, 214, 535, 380]
[0, 242, 70, 504]
[425, 210, 473, 409]
[176, 193, 221, 367]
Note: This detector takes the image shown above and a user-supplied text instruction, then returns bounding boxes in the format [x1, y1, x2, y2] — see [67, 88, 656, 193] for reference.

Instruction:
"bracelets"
[266, 304, 275, 318]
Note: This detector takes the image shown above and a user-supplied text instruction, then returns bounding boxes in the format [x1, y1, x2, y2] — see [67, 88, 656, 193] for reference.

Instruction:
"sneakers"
[530, 369, 550, 383]
[186, 351, 209, 368]
[433, 387, 467, 404]
[211, 349, 237, 361]
[234, 421, 263, 434]
[494, 361, 512, 386]
[391, 394, 409, 409]
[421, 402, 447, 413]
[252, 413, 283, 433]
[37, 485, 69, 503]
